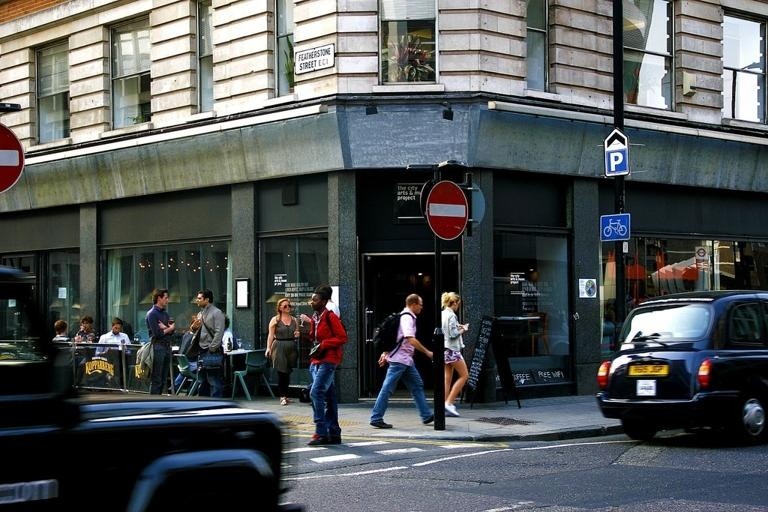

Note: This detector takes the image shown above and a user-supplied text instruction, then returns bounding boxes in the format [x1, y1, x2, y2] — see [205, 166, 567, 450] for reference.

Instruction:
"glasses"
[281, 305, 291, 308]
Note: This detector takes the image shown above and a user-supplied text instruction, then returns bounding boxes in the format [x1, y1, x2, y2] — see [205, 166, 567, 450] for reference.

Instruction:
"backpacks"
[373, 313, 415, 358]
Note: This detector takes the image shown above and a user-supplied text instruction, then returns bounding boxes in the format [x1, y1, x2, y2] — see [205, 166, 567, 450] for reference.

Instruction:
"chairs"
[515, 311, 550, 355]
[54, 342, 278, 400]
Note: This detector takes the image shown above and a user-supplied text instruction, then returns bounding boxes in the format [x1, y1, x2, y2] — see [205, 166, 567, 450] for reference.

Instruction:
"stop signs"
[426, 182, 469, 242]
[1, 124, 32, 195]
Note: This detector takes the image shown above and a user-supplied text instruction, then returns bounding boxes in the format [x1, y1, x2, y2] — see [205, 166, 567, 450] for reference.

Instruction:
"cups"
[169, 317, 175, 325]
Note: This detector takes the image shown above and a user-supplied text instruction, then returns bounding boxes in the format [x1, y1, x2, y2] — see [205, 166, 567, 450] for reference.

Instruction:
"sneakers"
[328, 438, 341, 445]
[286, 397, 294, 403]
[445, 403, 460, 416]
[370, 419, 392, 429]
[423, 414, 434, 424]
[280, 398, 287, 406]
[308, 438, 326, 445]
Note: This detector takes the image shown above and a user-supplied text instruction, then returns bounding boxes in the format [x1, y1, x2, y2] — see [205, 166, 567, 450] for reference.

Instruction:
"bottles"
[134, 336, 139, 344]
[227, 338, 233, 352]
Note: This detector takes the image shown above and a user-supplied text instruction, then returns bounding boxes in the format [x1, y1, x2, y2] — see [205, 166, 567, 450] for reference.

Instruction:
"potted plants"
[282, 35, 294, 93]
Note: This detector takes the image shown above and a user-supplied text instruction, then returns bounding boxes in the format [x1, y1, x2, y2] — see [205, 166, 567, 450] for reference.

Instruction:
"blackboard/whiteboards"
[518, 280, 555, 313]
[466, 315, 495, 391]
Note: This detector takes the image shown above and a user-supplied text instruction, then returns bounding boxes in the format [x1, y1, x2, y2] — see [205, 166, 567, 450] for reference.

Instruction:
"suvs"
[3, 263, 299, 512]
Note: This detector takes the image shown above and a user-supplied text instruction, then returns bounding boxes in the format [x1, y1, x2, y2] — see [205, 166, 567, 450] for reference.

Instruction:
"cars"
[592, 289, 768, 445]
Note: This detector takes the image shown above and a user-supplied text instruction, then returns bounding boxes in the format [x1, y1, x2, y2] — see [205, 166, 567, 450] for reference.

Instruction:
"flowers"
[382, 32, 435, 82]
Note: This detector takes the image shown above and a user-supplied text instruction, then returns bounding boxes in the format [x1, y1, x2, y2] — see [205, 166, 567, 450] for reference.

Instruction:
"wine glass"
[298, 315, 306, 327]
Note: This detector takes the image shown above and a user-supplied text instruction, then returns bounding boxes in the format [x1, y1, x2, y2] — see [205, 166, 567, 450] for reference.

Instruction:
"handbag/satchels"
[202, 354, 223, 369]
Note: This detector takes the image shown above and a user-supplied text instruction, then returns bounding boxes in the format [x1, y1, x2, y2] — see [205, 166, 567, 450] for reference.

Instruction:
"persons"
[189, 289, 225, 398]
[442, 290, 472, 419]
[53, 314, 239, 400]
[145, 287, 177, 394]
[367, 294, 436, 427]
[264, 298, 301, 406]
[305, 284, 347, 443]
[299, 285, 343, 406]
